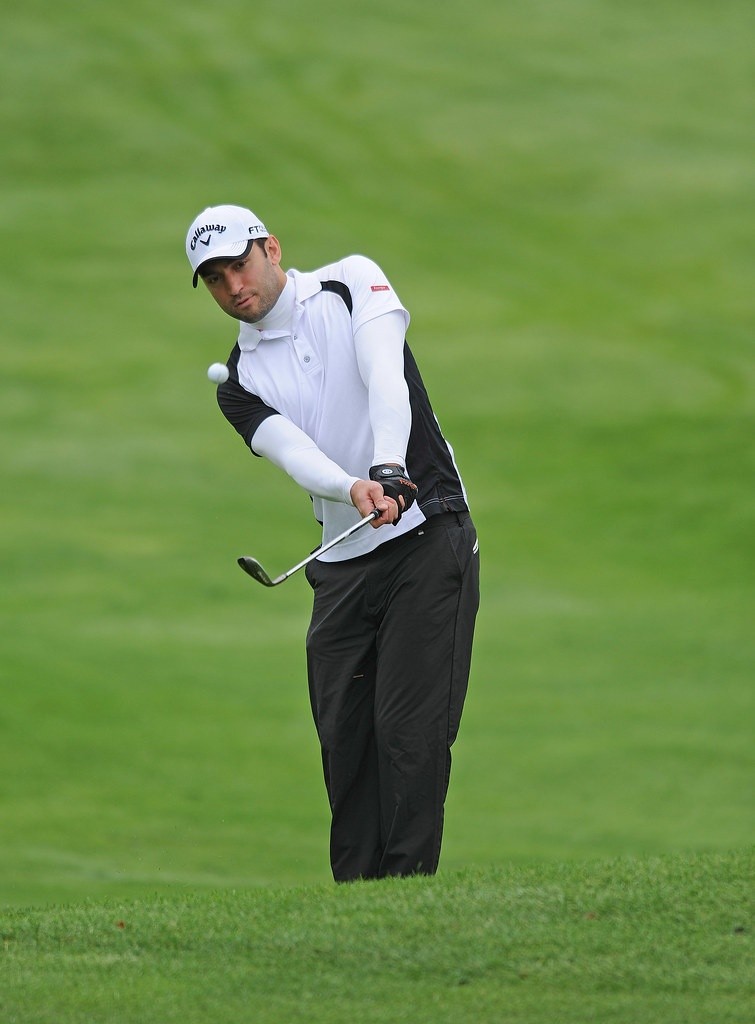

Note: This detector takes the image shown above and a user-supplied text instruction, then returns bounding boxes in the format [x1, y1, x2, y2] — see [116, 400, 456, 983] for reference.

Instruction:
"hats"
[185, 205, 269, 288]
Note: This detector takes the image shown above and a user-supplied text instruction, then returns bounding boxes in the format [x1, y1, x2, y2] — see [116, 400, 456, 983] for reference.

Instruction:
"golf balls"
[206, 361, 230, 384]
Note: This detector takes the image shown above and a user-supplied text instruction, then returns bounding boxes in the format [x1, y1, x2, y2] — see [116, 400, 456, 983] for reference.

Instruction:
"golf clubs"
[236, 508, 383, 588]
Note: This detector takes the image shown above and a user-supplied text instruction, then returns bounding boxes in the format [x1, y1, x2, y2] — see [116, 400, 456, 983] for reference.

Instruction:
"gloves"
[369, 464, 418, 526]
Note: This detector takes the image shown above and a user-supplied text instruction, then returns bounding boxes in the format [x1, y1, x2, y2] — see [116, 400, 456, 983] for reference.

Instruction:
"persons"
[184, 206, 481, 884]
